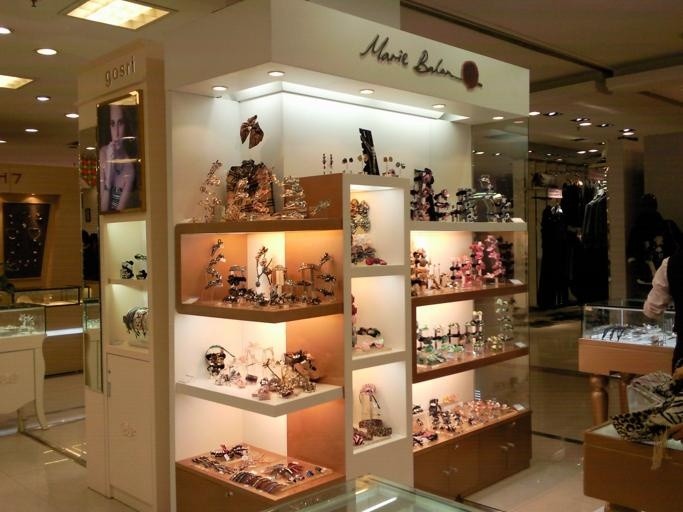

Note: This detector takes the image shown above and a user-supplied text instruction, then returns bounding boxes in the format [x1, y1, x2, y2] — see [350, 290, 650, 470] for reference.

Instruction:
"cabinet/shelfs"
[2, 303, 50, 433]
[529, 185, 683, 511]
[8, 278, 84, 377]
[78, 3, 532, 510]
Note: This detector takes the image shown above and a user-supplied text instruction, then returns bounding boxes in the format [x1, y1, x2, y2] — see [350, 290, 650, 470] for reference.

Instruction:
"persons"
[642, 255, 683, 395]
[97, 101, 135, 214]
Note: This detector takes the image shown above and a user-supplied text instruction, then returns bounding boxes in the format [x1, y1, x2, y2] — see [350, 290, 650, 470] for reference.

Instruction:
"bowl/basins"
[112, 154, 126, 175]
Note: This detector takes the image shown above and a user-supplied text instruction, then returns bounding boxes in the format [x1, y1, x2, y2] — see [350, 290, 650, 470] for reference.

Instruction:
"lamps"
[67, 1, 174, 30]
[1, 74, 34, 90]
